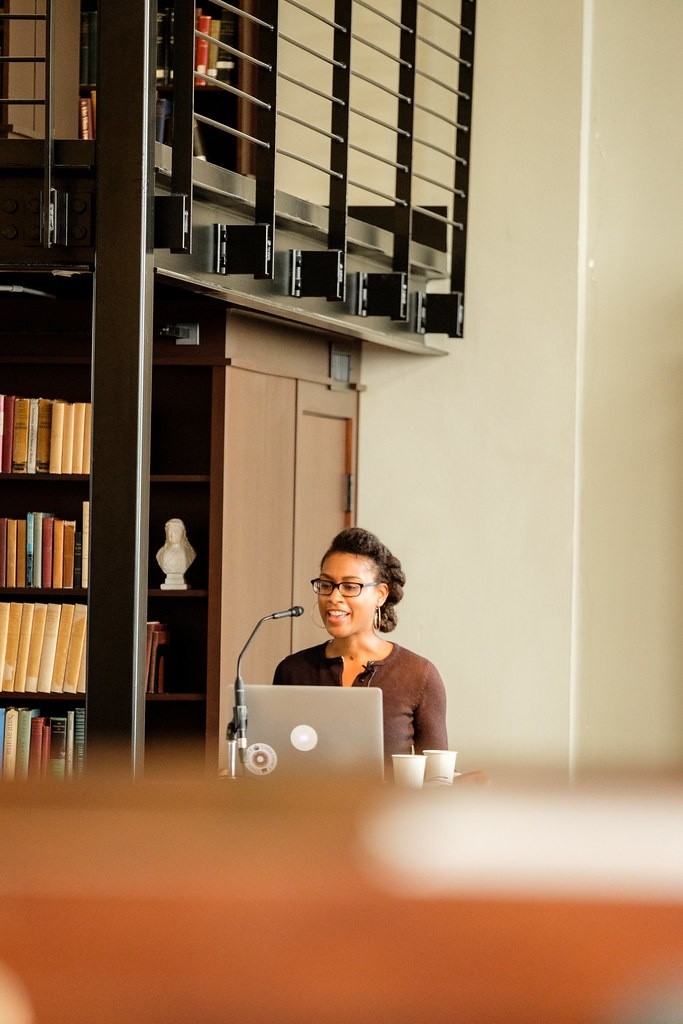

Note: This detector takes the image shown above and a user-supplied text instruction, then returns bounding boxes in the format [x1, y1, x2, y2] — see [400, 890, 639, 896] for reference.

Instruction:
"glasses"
[310, 578, 380, 597]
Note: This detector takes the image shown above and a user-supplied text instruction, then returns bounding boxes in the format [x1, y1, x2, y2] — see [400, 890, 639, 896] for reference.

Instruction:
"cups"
[391, 754, 428, 788]
[423, 749, 459, 785]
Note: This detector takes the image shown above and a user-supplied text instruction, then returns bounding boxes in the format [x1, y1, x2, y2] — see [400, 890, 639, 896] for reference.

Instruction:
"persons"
[272, 530, 448, 783]
[155, 519, 197, 574]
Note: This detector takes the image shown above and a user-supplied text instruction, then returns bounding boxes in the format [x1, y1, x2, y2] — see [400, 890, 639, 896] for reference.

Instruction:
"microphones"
[234, 607, 305, 766]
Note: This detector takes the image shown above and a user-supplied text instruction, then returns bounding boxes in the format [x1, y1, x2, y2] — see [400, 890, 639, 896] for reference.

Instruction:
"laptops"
[227, 682, 384, 785]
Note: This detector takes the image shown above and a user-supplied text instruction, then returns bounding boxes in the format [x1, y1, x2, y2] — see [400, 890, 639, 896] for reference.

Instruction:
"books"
[0, 501, 90, 588]
[146, 621, 171, 695]
[0, 707, 86, 784]
[78, 7, 235, 162]
[0, 602, 87, 693]
[0, 394, 92, 475]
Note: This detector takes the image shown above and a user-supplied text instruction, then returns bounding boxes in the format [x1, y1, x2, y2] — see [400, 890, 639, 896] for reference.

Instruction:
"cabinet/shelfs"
[77, 0, 258, 179]
[0, 302, 367, 796]
